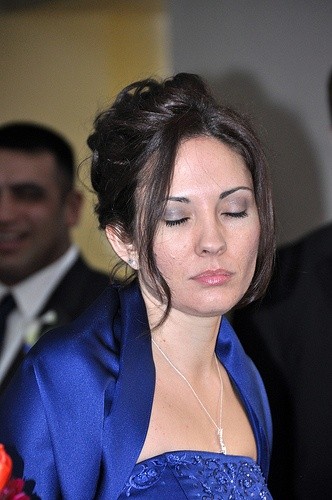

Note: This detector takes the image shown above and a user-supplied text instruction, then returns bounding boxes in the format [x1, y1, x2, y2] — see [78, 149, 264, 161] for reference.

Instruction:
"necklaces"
[150, 335, 227, 456]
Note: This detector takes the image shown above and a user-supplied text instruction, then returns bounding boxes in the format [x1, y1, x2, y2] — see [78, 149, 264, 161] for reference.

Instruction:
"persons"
[0, 73, 277, 499]
[225, 68, 332, 500]
[0, 120, 128, 398]
